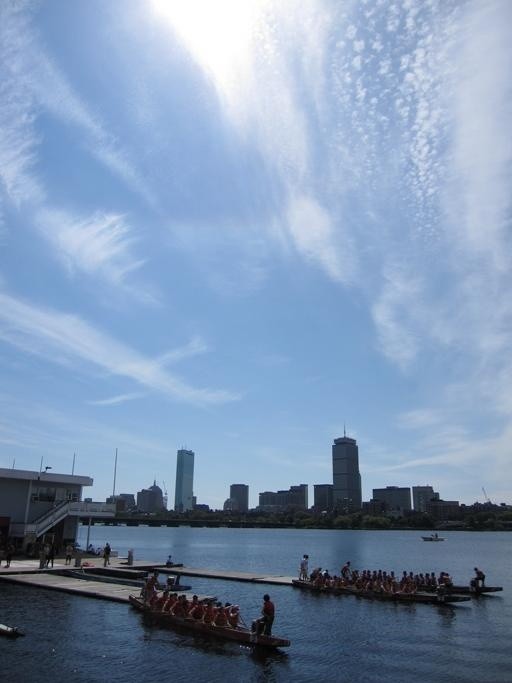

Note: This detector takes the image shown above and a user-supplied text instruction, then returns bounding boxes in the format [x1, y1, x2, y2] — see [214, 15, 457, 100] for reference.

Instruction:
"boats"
[0, 622, 26, 638]
[128, 592, 292, 647]
[338, 574, 504, 594]
[420, 535, 449, 541]
[291, 578, 471, 603]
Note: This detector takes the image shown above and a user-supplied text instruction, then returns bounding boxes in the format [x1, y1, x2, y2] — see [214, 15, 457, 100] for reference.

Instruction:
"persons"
[166, 554, 174, 564]
[298, 552, 454, 607]
[141, 572, 240, 629]
[471, 568, 486, 586]
[0, 539, 15, 568]
[431, 532, 439, 538]
[33, 539, 55, 569]
[64, 543, 74, 564]
[251, 593, 275, 636]
[88, 542, 111, 567]
